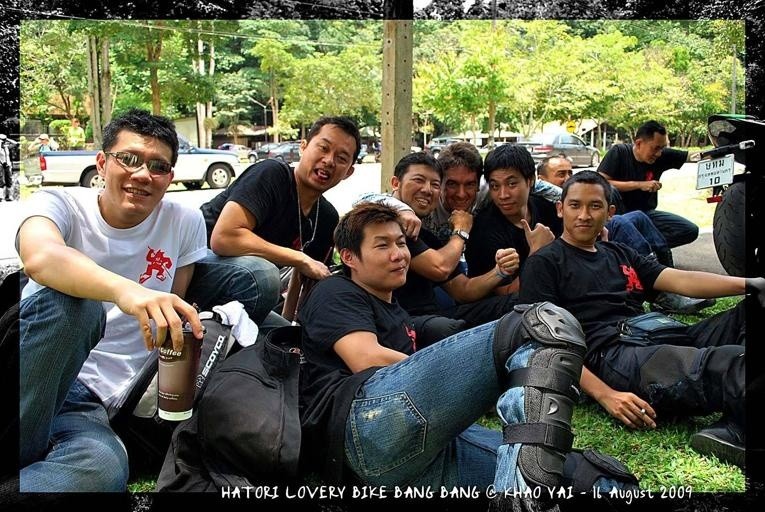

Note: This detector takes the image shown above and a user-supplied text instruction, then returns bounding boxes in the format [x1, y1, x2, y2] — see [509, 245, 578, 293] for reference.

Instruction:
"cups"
[154, 325, 204, 422]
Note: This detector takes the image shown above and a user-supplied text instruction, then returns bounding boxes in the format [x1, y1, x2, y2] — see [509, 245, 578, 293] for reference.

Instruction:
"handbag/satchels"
[615, 311, 692, 347]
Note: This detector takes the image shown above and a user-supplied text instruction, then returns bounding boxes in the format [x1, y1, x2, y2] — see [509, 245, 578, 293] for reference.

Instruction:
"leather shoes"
[691, 418, 745, 468]
[650, 290, 717, 314]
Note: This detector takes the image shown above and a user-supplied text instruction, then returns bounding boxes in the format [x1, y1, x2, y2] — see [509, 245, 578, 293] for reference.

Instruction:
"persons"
[66, 118, 85, 149]
[0, 132, 18, 202]
[182, 115, 763, 508]
[28, 134, 59, 156]
[0, 107, 207, 510]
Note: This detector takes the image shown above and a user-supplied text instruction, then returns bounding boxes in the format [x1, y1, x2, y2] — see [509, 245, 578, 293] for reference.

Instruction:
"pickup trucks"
[39, 132, 242, 190]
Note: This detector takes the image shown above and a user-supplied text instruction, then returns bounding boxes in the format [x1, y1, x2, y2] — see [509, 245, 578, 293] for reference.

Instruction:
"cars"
[515, 133, 600, 167]
[233, 135, 467, 163]
[483, 142, 509, 150]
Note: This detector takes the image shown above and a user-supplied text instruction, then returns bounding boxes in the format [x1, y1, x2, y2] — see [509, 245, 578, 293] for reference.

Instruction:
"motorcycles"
[690, 114, 765, 279]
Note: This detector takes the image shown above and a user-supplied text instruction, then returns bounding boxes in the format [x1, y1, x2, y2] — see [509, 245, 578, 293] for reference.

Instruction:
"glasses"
[104, 151, 173, 176]
